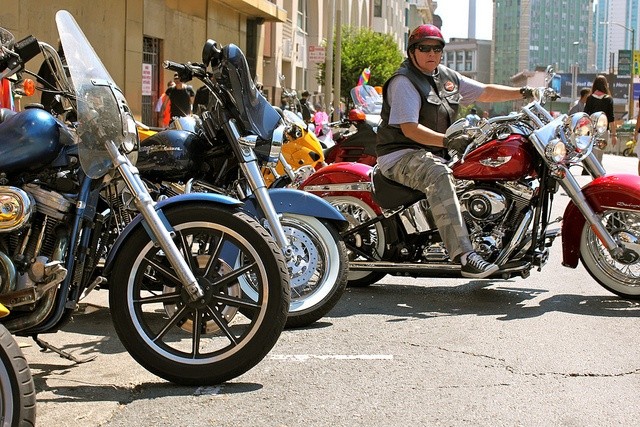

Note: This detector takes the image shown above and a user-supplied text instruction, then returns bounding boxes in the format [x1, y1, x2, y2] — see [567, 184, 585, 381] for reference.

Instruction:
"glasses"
[416, 45, 443, 53]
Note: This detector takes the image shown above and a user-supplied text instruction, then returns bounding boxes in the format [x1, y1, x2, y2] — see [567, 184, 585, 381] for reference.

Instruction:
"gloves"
[444, 132, 473, 154]
[520, 86, 561, 102]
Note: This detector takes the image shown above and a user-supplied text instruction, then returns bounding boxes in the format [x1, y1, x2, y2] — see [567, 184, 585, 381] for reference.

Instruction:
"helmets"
[348, 109, 366, 122]
[406, 25, 445, 59]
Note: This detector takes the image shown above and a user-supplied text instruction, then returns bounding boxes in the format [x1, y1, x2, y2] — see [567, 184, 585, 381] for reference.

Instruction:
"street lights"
[570, 41, 579, 104]
[599, 21, 635, 120]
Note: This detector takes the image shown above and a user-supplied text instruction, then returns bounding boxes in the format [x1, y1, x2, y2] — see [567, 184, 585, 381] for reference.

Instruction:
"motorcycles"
[296, 63, 640, 302]
[0, 9, 290, 385]
[0, 38, 349, 329]
[259, 151, 315, 188]
[323, 84, 382, 167]
[623, 129, 636, 156]
[136, 104, 330, 188]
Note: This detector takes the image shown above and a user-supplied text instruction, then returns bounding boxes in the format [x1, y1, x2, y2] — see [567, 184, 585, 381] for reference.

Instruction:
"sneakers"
[460, 251, 499, 278]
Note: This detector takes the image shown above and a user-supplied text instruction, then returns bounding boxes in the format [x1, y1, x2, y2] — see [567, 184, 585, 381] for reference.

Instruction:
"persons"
[155, 81, 176, 127]
[376, 23, 553, 277]
[300, 91, 316, 122]
[565, 88, 592, 175]
[159, 72, 195, 127]
[0, 73, 21, 112]
[308, 103, 333, 139]
[36, 36, 76, 117]
[633, 95, 640, 179]
[584, 74, 618, 164]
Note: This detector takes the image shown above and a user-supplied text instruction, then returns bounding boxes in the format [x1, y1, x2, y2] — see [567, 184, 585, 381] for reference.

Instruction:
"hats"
[173, 72, 179, 80]
[301, 91, 312, 98]
